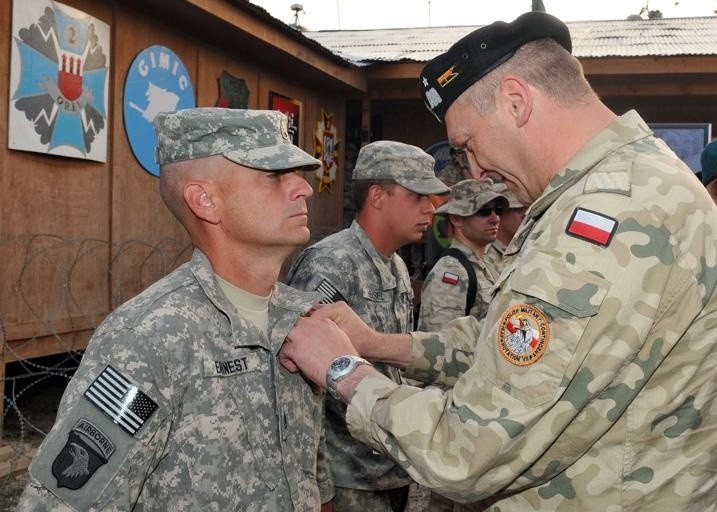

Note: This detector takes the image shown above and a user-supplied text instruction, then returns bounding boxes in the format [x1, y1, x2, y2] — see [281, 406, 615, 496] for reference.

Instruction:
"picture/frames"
[267, 89, 304, 177]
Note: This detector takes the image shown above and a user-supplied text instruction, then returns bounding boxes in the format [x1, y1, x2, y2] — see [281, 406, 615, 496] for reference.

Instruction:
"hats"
[434, 178, 509, 217]
[420, 11, 572, 128]
[154, 107, 322, 171]
[352, 141, 452, 195]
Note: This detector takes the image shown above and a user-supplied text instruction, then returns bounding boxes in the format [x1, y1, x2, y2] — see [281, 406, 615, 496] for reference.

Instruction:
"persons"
[699, 138, 716, 204]
[408, 178, 510, 510]
[278, 10, 717, 510]
[13, 106, 340, 510]
[482, 190, 532, 282]
[285, 138, 452, 511]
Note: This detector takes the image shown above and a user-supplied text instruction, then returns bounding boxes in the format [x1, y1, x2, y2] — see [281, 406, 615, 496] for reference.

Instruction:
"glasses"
[480, 204, 503, 216]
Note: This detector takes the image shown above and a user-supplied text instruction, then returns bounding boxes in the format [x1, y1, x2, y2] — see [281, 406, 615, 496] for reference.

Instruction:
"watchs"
[325, 355, 372, 402]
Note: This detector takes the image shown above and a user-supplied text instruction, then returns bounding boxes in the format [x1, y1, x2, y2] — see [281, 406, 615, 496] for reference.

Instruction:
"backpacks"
[413, 248, 476, 329]
